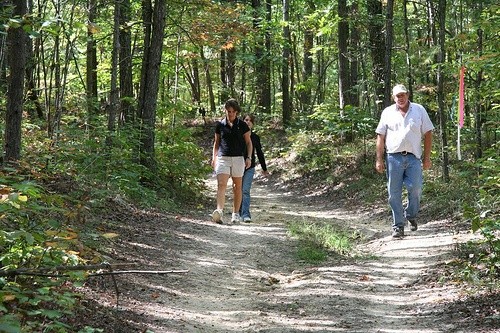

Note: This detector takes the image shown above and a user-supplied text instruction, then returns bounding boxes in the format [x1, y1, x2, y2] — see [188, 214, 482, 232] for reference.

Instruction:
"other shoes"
[393, 228, 404, 237]
[243, 217, 251, 222]
[408, 220, 417, 231]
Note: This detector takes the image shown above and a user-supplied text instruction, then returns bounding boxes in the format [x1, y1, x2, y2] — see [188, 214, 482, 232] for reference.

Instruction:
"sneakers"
[231, 213, 240, 224]
[213, 209, 223, 224]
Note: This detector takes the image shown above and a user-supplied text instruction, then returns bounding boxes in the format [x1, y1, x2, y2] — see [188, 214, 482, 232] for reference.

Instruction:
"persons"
[229, 114, 269, 222]
[210, 98, 252, 223]
[375, 85, 435, 237]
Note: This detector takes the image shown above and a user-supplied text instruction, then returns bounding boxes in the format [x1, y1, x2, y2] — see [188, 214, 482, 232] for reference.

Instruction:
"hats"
[392, 84, 407, 96]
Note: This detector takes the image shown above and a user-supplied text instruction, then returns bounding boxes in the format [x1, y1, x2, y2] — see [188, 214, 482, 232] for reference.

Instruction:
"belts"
[395, 151, 413, 155]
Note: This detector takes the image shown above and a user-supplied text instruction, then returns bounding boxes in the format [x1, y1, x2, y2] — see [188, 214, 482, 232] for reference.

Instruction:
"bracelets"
[246, 157, 252, 160]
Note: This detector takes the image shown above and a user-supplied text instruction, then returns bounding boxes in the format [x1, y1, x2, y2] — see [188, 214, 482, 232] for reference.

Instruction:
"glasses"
[227, 110, 236, 113]
